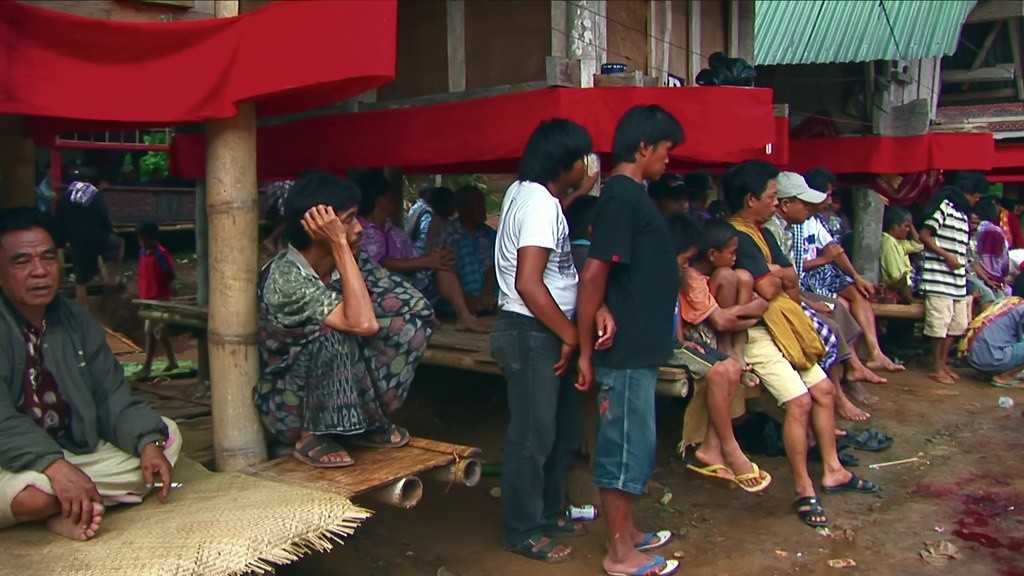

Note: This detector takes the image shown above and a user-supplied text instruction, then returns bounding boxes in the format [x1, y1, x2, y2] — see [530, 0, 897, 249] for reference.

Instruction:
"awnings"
[754, 0, 979, 67]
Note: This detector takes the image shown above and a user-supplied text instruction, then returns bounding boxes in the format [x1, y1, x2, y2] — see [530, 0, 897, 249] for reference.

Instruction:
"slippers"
[637, 530, 672, 549]
[837, 453, 859, 467]
[821, 472, 878, 495]
[990, 380, 1021, 387]
[732, 463, 772, 492]
[687, 463, 735, 482]
[794, 496, 829, 527]
[840, 427, 894, 452]
[342, 423, 410, 448]
[606, 554, 680, 576]
[293, 436, 355, 468]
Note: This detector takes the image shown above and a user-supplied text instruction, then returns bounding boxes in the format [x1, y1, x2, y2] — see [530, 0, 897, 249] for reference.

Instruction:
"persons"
[675, 158, 906, 529]
[356, 176, 494, 334]
[0, 212, 183, 541]
[876, 171, 1024, 388]
[572, 104, 680, 576]
[130, 219, 179, 381]
[62, 160, 126, 300]
[491, 118, 616, 562]
[250, 171, 435, 468]
[410, 185, 498, 318]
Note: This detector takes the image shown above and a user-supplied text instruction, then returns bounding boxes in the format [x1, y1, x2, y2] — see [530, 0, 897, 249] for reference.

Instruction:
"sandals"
[541, 514, 587, 537]
[505, 535, 575, 561]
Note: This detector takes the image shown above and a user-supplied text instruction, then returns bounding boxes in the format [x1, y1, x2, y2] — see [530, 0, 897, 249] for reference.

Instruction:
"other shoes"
[947, 371, 961, 381]
[928, 373, 954, 385]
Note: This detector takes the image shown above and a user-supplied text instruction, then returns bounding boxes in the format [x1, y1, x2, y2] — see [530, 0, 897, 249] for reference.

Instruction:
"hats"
[775, 171, 827, 203]
